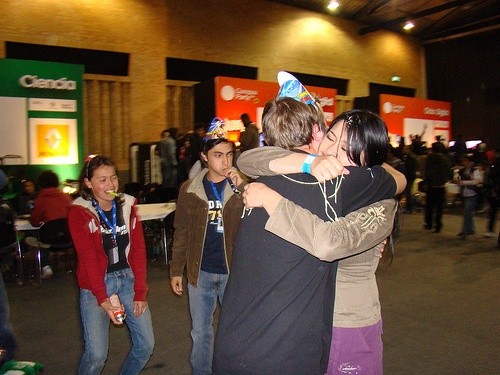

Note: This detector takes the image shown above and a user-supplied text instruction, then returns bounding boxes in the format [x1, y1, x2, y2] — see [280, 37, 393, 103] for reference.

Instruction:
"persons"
[30, 170, 73, 279]
[155, 110, 500, 254]
[68, 154, 154, 375]
[169, 139, 249, 375]
[211, 93, 406, 375]
[236, 109, 398, 375]
[0, 167, 46, 375]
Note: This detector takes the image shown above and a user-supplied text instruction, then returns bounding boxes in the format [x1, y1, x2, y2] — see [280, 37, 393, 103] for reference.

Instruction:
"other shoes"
[456, 230, 475, 240]
[424, 224, 431, 229]
[481, 231, 496, 238]
[433, 223, 444, 232]
[42, 265, 54, 280]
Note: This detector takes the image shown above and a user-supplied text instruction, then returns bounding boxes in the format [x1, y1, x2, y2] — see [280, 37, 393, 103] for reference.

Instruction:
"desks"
[15, 204, 176, 271]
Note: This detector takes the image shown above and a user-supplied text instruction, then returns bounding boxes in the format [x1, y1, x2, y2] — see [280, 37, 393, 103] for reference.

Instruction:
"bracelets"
[301, 154, 318, 174]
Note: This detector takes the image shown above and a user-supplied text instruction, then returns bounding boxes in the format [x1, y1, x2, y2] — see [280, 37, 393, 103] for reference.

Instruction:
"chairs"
[36, 217, 75, 284]
